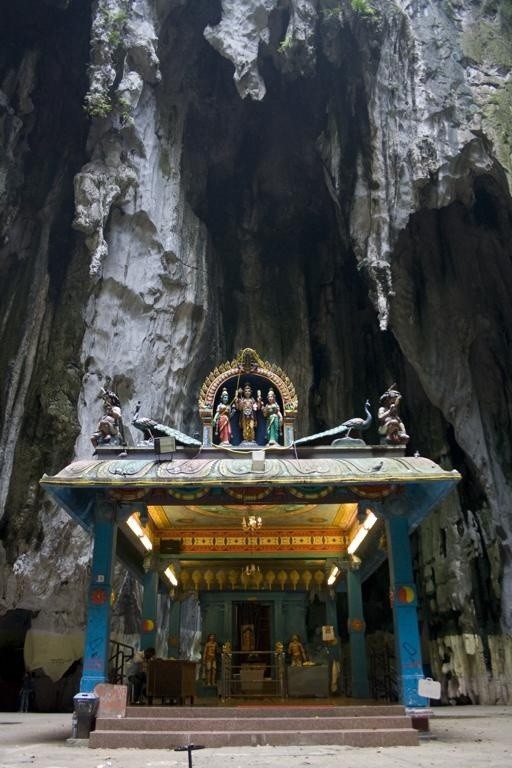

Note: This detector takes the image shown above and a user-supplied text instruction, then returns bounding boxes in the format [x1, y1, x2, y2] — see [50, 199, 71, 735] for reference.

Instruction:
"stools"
[126, 679, 148, 704]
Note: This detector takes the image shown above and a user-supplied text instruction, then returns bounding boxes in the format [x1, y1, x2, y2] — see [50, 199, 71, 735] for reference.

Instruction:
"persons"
[209, 385, 236, 450]
[376, 385, 410, 442]
[126, 645, 158, 706]
[238, 383, 260, 446]
[203, 634, 219, 682]
[287, 634, 309, 666]
[92, 387, 121, 446]
[262, 388, 282, 448]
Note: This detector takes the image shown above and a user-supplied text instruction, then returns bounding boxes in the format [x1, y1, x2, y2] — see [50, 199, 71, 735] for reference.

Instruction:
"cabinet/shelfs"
[223, 648, 286, 701]
[144, 656, 196, 705]
